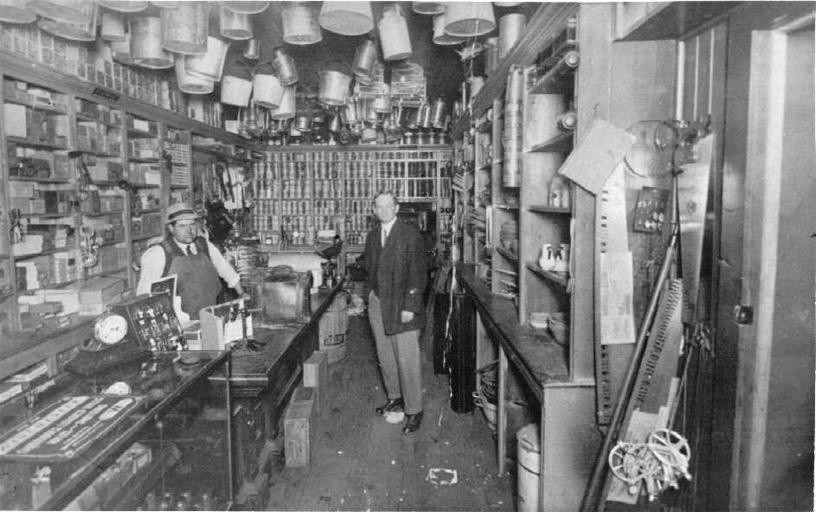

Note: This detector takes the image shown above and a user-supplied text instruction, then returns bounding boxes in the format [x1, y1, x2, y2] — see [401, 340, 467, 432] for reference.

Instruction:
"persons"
[138, 202, 253, 327]
[340, 191, 429, 434]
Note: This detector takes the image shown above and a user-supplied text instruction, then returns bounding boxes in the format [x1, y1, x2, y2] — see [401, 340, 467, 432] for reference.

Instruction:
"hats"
[162, 202, 203, 226]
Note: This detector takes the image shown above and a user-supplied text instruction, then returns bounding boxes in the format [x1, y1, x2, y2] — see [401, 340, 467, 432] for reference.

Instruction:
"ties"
[186, 245, 193, 255]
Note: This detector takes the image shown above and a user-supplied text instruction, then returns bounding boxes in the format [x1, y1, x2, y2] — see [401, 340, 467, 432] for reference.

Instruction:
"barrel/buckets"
[515, 421, 542, 512]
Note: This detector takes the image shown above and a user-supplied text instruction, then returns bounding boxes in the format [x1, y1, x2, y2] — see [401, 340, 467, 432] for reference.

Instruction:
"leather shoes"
[377, 398, 404, 416]
[403, 410, 424, 434]
